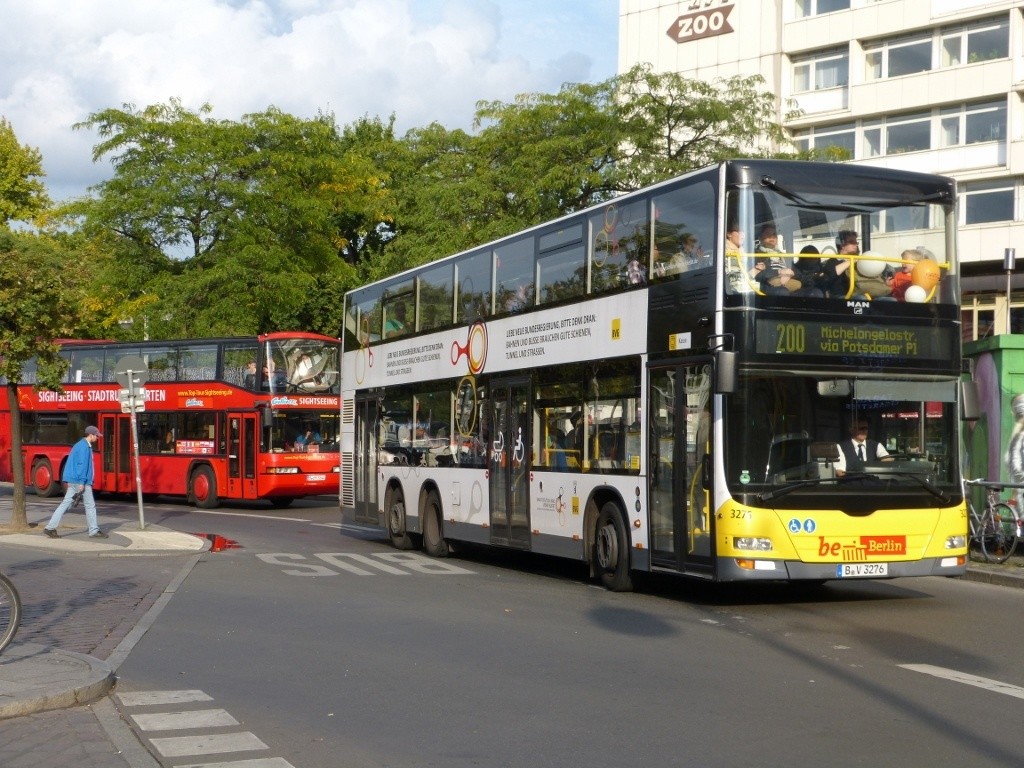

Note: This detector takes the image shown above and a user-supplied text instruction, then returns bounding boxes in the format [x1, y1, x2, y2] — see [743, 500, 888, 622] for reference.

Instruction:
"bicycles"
[960, 476, 1022, 565]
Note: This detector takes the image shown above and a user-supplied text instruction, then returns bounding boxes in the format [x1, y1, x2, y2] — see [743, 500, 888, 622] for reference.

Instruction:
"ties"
[857, 444, 864, 462]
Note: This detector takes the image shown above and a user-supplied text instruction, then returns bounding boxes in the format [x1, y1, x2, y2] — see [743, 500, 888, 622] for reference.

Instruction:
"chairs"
[141, 439, 164, 452]
[0, 363, 249, 386]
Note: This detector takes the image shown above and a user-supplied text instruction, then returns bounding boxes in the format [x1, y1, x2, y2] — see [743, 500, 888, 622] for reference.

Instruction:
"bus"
[333, 155, 971, 595]
[0, 329, 340, 509]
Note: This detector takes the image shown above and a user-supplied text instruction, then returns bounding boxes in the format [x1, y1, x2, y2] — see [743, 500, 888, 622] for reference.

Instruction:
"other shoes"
[89, 531, 108, 539]
[43, 529, 63, 539]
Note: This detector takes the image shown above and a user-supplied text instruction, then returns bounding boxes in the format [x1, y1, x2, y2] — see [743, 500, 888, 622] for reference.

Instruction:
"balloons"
[912, 259, 940, 289]
[855, 249, 886, 278]
[905, 286, 925, 304]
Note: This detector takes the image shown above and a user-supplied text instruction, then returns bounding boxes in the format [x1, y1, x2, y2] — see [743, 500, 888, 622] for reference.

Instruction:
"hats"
[84, 426, 104, 437]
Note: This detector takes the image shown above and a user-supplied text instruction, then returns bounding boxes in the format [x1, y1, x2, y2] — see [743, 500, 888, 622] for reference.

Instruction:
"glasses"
[858, 426, 867, 430]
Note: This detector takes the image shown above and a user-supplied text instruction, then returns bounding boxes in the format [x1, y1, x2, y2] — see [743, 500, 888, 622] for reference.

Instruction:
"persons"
[381, 223, 932, 339]
[397, 414, 897, 479]
[158, 361, 322, 455]
[42, 426, 108, 538]
